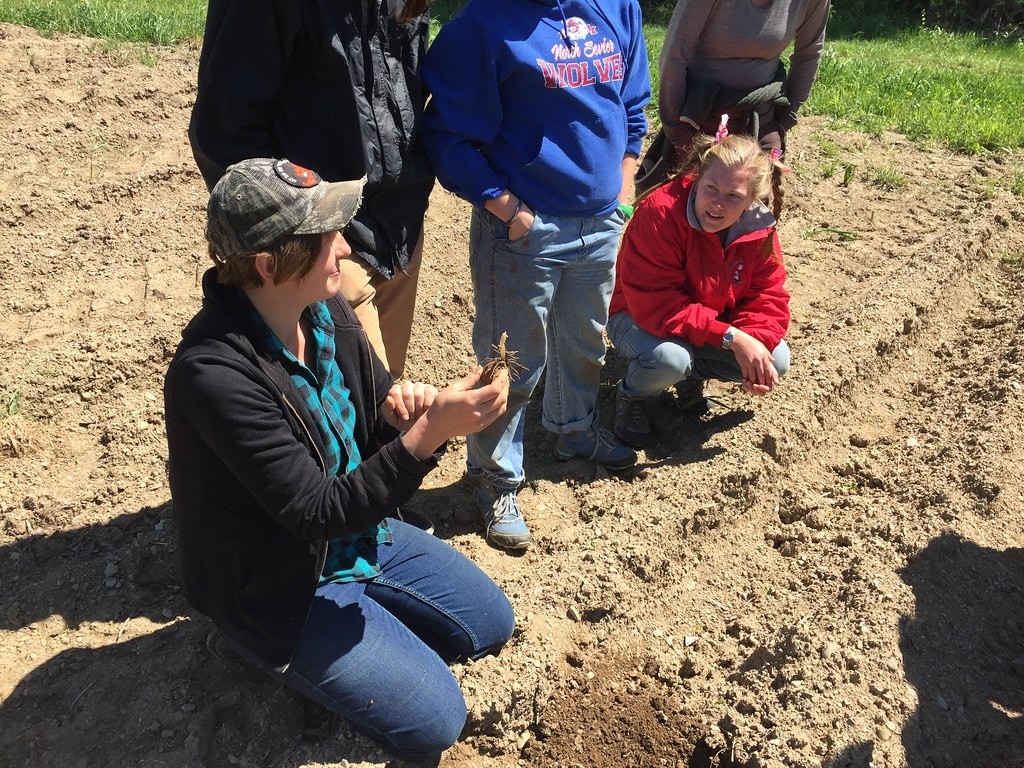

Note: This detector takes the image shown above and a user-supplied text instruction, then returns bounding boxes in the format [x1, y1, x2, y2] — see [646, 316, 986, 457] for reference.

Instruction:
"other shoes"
[206, 627, 269, 683]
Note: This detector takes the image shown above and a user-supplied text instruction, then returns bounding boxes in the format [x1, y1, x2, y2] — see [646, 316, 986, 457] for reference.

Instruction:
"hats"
[206, 158, 368, 262]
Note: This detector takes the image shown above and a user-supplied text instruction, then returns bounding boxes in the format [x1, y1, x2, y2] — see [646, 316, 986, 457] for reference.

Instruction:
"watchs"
[722, 326, 736, 350]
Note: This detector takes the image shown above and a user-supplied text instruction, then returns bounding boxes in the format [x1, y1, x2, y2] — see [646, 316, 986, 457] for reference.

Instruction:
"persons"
[163, 160, 517, 754]
[187, 1, 436, 375]
[421, 0, 652, 550]
[611, 134, 790, 448]
[634, 1, 831, 196]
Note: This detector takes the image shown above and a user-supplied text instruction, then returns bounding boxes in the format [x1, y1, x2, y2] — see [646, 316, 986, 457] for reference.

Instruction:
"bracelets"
[505, 199, 523, 226]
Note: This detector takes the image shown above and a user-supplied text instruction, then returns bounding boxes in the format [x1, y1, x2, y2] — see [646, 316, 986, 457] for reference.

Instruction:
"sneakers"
[473, 478, 531, 548]
[613, 376, 652, 447]
[554, 425, 638, 469]
[673, 369, 712, 412]
